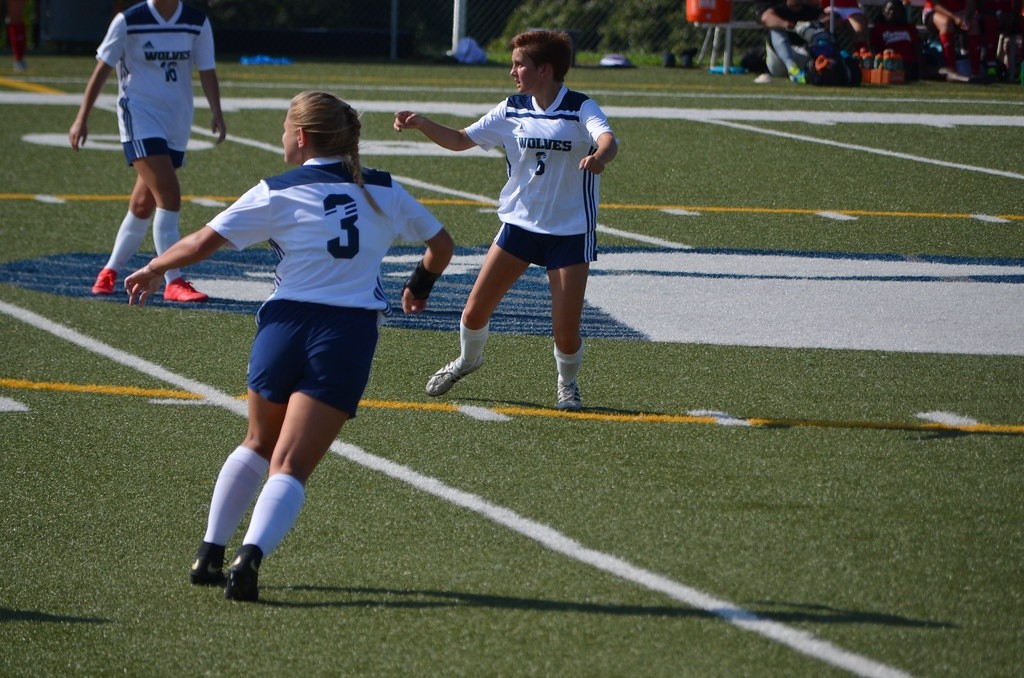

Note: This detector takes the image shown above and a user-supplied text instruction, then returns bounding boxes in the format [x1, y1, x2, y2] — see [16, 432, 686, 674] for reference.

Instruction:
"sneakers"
[557, 376, 581, 411]
[425, 354, 484, 396]
[163, 280, 209, 302]
[223, 544, 264, 601]
[190, 541, 229, 586]
[91, 268, 116, 294]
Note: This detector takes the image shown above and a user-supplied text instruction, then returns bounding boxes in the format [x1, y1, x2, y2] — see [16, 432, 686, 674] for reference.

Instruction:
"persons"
[754, 0, 1024, 85]
[123, 90, 456, 603]
[0, 0, 32, 73]
[69, 0, 229, 304]
[394, 27, 619, 410]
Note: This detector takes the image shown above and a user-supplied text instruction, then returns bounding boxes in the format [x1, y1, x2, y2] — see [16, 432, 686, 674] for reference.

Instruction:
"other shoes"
[946, 72, 969, 82]
[789, 66, 808, 83]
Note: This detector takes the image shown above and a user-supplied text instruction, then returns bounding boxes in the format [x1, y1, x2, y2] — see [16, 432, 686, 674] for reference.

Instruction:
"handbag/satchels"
[806, 50, 863, 87]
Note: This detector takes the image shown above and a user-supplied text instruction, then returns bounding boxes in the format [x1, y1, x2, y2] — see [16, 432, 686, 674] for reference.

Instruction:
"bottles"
[854, 47, 903, 72]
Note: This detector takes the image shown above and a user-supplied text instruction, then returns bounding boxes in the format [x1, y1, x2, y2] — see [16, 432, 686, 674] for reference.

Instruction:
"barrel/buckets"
[686, 0, 733, 23]
